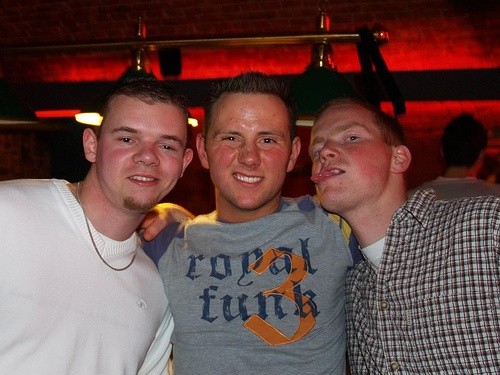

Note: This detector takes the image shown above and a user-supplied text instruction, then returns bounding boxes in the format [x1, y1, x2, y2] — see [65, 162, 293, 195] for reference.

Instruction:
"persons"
[143, 73, 365, 375]
[0, 79, 193, 375]
[408, 114, 500, 199]
[135, 96, 500, 375]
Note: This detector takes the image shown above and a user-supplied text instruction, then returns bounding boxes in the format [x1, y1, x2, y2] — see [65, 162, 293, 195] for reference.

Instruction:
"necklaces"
[77, 181, 141, 272]
